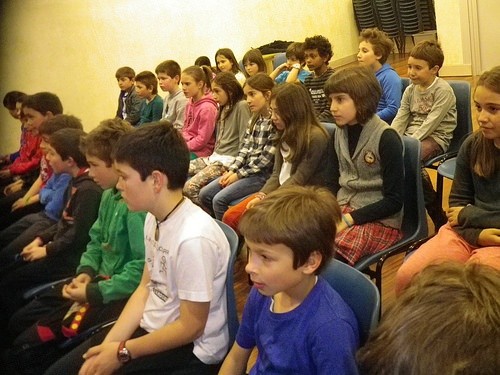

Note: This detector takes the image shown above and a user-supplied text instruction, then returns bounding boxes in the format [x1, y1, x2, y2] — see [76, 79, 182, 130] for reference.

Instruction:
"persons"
[0, 28, 500, 375]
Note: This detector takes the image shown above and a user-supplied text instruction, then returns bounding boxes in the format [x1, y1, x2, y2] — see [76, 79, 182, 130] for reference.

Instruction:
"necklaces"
[155, 197, 184, 241]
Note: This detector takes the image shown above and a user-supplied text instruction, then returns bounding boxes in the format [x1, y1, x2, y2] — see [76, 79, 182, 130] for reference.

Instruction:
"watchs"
[117, 339, 132, 367]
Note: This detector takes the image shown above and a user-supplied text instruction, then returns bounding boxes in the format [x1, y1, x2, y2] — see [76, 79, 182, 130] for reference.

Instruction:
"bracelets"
[291, 64, 301, 74]
[24, 197, 30, 205]
[341, 214, 352, 228]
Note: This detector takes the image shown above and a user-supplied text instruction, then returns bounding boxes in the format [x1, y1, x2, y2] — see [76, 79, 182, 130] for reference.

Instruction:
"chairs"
[15, 0, 473, 374]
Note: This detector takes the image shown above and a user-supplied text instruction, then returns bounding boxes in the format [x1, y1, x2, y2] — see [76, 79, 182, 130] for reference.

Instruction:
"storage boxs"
[412, 30, 437, 45]
